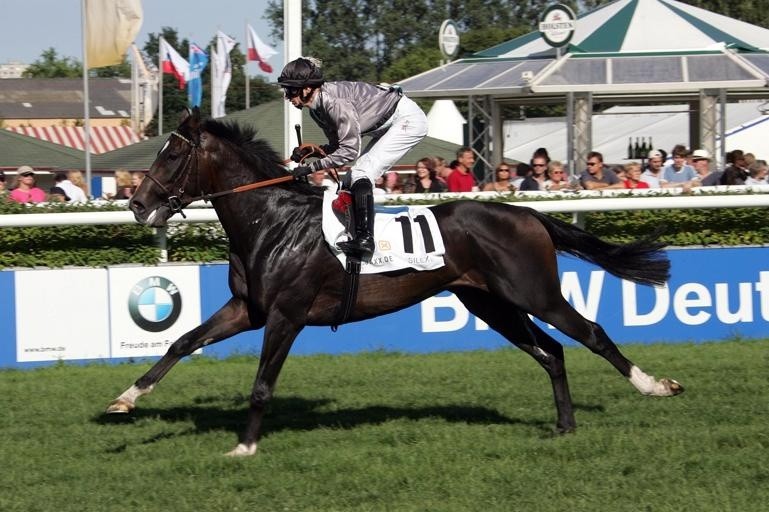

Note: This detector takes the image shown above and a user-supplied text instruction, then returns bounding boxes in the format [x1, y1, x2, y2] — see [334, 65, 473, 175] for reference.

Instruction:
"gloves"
[291, 146, 312, 163]
[291, 164, 312, 176]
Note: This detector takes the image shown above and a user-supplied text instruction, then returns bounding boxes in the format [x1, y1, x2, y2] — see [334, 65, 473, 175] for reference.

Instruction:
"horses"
[105, 105, 685, 458]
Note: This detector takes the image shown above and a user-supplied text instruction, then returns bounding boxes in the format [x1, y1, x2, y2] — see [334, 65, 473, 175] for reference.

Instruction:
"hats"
[691, 150, 710, 159]
[271, 59, 322, 87]
[648, 151, 662, 158]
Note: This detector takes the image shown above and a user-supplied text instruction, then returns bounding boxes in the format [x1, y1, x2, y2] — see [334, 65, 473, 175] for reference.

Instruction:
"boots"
[336, 179, 375, 255]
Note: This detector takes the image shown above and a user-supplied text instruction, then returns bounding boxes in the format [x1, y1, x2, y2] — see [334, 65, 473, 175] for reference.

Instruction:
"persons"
[0, 165, 145, 203]
[374, 148, 478, 198]
[482, 145, 769, 191]
[328, 168, 343, 191]
[308, 170, 334, 188]
[278, 57, 429, 255]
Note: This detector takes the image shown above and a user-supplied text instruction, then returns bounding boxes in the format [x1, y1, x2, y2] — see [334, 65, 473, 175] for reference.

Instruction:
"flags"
[85, 0, 279, 134]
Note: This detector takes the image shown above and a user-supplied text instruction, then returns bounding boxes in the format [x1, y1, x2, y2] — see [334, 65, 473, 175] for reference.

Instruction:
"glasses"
[500, 162, 595, 174]
